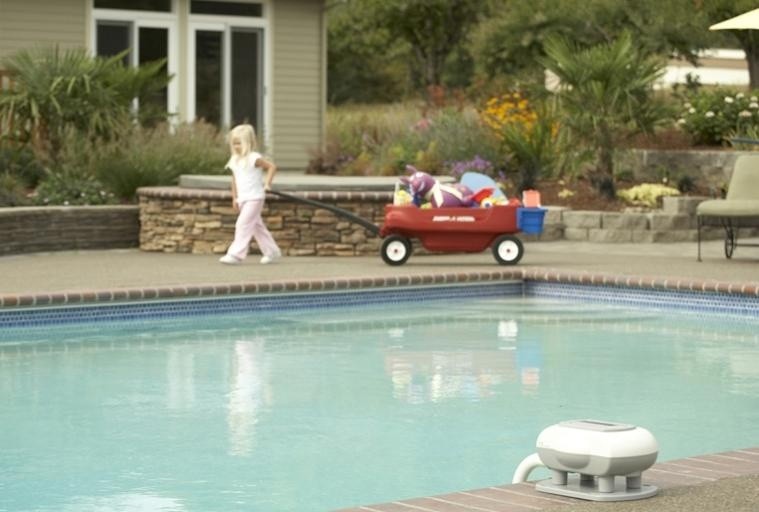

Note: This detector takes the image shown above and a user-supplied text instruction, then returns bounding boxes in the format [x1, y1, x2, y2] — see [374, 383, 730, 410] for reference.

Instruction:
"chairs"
[219, 255, 240, 264]
[260, 248, 281, 263]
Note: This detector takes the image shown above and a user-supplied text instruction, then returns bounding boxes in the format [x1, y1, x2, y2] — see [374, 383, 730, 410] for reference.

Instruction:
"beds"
[709, 8, 759, 32]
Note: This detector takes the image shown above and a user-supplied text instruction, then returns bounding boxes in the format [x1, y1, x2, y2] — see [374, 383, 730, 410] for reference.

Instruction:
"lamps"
[696, 153, 759, 261]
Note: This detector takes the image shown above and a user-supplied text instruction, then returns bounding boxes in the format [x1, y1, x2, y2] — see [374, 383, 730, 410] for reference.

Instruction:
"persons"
[219, 123, 282, 264]
